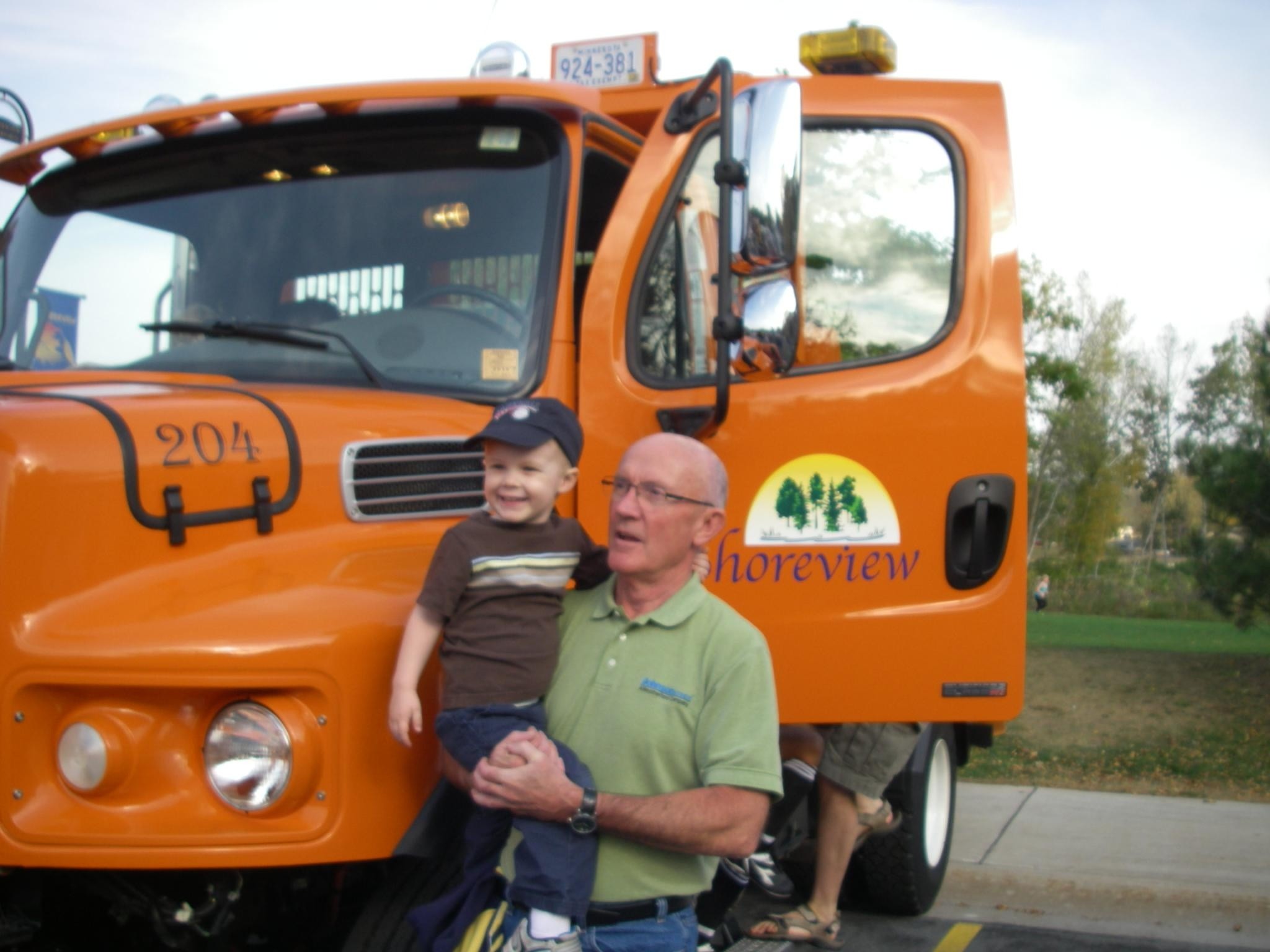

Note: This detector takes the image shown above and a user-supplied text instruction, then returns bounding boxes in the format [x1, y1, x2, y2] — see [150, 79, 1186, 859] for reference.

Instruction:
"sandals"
[747, 903, 846, 951]
[854, 798, 903, 850]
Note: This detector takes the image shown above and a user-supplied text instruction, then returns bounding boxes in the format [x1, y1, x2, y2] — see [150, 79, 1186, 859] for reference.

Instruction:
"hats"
[461, 397, 583, 466]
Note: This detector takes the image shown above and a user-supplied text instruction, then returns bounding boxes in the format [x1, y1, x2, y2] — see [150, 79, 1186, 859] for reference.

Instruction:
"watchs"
[565, 786, 600, 833]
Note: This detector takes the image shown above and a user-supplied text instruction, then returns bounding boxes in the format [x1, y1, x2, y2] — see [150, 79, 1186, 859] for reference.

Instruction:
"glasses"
[600, 474, 713, 509]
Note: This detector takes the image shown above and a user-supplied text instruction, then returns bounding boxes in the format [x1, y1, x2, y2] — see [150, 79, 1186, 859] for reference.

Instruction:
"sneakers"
[502, 918, 584, 952]
[722, 853, 795, 899]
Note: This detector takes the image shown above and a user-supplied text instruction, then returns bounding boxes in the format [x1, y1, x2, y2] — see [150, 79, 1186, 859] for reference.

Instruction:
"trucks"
[0, 34, 1029, 952]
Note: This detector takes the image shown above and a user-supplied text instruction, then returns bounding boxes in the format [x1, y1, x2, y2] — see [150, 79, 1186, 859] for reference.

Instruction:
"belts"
[574, 897, 696, 925]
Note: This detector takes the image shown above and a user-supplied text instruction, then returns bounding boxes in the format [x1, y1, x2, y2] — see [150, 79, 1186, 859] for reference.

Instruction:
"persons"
[385, 392, 710, 952]
[434, 426, 786, 952]
[743, 718, 926, 945]
